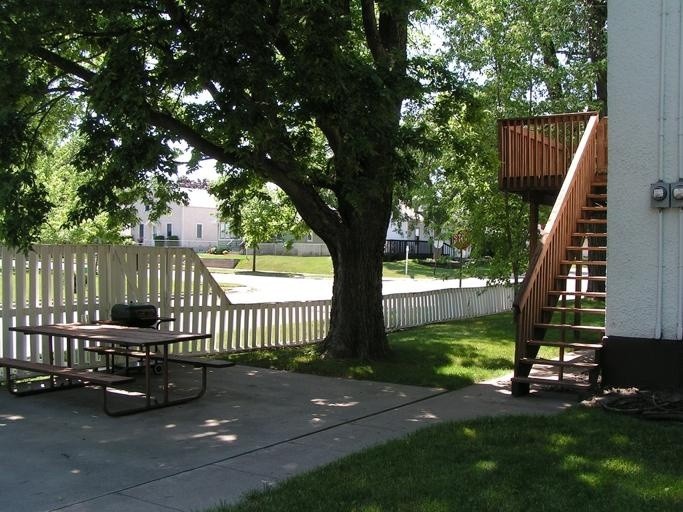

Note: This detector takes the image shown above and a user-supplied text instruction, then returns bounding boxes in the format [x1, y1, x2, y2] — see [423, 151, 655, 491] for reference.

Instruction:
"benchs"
[0, 357, 136, 417]
[84, 346, 235, 396]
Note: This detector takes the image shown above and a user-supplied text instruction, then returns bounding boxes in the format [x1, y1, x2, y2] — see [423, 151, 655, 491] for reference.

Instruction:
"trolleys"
[93, 318, 176, 374]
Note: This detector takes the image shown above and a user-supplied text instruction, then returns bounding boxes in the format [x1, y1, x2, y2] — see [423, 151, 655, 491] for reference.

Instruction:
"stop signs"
[452, 230, 468, 249]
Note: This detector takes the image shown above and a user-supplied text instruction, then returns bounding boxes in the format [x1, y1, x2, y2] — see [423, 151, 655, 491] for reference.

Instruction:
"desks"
[8, 319, 212, 408]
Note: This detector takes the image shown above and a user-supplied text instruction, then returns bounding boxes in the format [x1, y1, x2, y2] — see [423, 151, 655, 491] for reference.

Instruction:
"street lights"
[415, 226, 420, 258]
